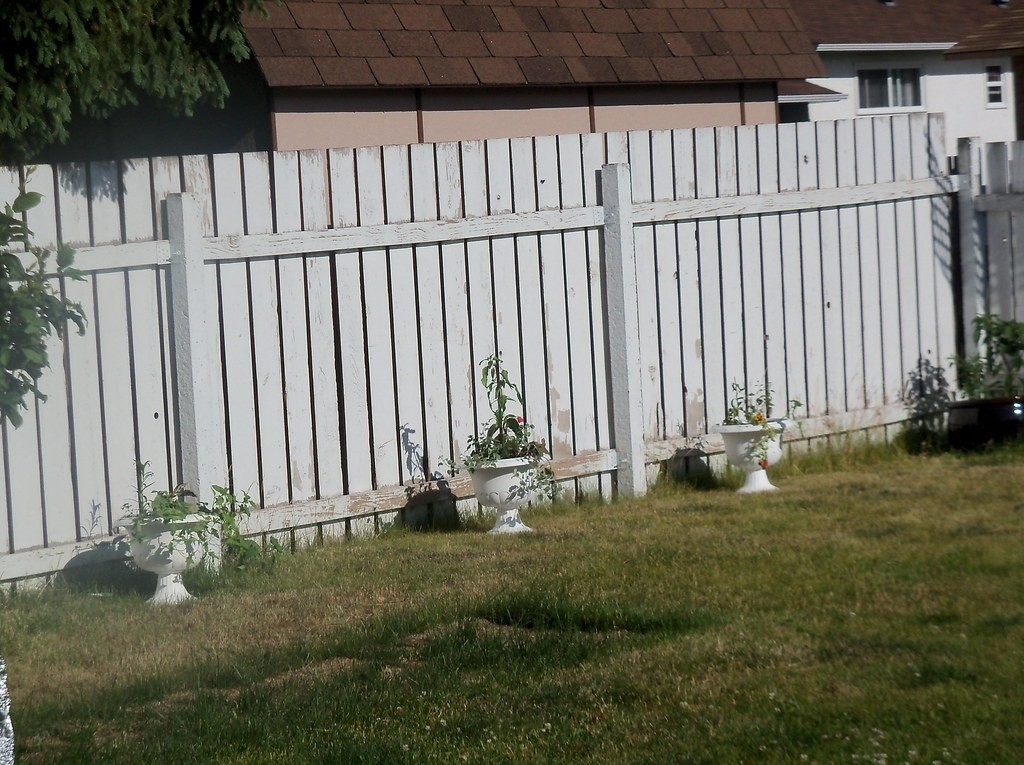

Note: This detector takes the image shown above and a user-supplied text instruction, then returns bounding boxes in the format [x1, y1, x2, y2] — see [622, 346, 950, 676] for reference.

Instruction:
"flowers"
[722, 377, 809, 469]
[436, 350, 563, 502]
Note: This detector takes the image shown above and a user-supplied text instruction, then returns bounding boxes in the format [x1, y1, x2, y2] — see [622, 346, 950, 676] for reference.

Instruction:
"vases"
[464, 454, 554, 535]
[709, 418, 795, 493]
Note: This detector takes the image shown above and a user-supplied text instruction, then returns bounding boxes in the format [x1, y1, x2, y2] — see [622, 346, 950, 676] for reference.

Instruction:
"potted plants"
[117, 459, 283, 608]
[946, 313, 1024, 446]
